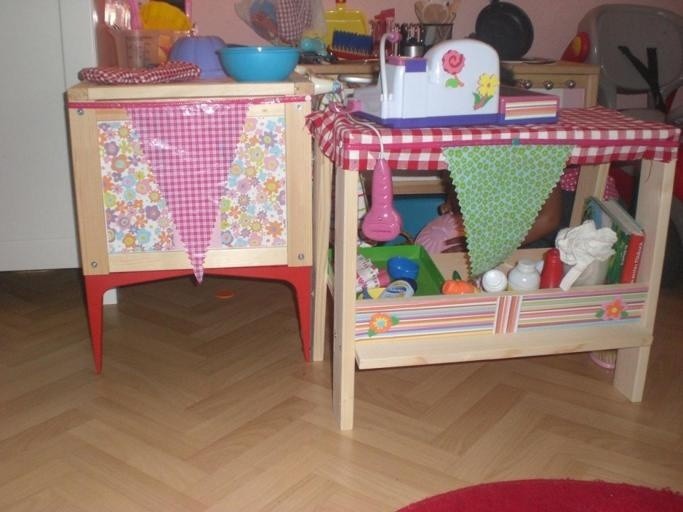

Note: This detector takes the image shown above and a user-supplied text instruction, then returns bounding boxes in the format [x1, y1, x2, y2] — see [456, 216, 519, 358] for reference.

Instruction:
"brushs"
[326, 29, 377, 61]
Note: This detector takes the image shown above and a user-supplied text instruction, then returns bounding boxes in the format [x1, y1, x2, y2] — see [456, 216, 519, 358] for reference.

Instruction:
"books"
[580, 196, 644, 285]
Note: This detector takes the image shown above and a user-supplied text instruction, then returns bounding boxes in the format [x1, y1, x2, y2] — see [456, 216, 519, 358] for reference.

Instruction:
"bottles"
[482, 248, 564, 292]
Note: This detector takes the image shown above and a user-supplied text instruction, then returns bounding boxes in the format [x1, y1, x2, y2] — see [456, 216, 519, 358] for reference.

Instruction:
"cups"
[112, 28, 160, 70]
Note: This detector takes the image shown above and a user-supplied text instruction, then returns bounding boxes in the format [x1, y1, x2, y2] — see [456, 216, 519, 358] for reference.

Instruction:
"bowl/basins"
[169, 36, 226, 80]
[215, 47, 301, 84]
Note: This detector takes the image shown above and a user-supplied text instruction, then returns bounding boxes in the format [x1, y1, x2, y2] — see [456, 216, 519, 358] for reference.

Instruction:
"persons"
[437, 162, 624, 254]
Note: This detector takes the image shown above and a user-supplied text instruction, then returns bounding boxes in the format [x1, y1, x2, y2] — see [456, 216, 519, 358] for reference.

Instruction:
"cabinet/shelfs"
[66, 70, 316, 376]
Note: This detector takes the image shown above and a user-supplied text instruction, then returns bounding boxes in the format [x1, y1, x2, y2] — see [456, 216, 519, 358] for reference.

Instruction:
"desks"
[295, 58, 602, 111]
[309, 107, 681, 431]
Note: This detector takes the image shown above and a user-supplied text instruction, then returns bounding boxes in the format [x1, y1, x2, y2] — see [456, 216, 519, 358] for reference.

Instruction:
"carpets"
[392, 479, 683, 512]
